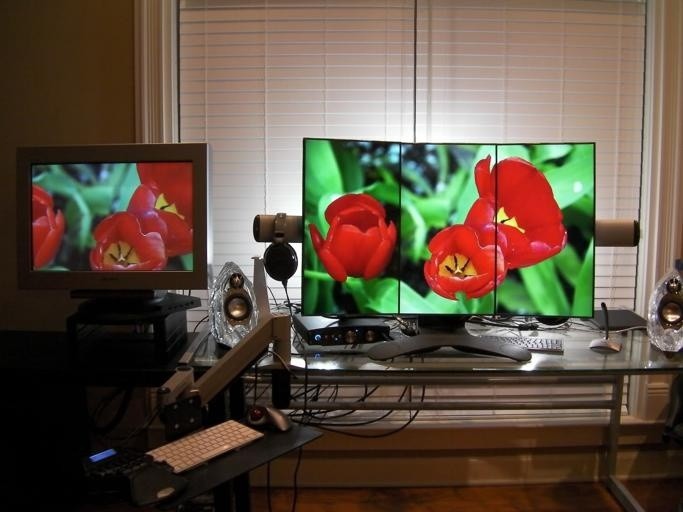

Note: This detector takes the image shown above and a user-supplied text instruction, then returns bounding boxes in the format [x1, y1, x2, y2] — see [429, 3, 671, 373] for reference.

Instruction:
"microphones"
[589, 302, 622, 353]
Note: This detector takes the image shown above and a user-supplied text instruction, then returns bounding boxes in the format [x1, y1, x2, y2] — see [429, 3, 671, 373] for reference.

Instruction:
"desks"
[178, 310, 683, 512]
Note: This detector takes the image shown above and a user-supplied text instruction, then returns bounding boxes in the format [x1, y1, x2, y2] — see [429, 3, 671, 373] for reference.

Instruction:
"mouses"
[247, 406, 292, 432]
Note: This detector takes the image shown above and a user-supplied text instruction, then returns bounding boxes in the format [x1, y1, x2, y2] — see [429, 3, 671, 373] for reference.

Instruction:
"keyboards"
[145, 419, 264, 473]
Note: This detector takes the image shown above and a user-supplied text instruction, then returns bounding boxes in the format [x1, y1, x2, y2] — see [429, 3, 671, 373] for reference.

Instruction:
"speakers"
[209, 262, 259, 349]
[647, 272, 683, 353]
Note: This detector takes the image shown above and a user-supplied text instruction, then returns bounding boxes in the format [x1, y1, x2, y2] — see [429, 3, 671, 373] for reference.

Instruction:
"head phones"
[264, 213, 297, 280]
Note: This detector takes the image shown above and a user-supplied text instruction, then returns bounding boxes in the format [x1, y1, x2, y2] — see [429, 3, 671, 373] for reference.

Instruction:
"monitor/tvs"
[15, 142, 206, 309]
[301, 139, 596, 362]
